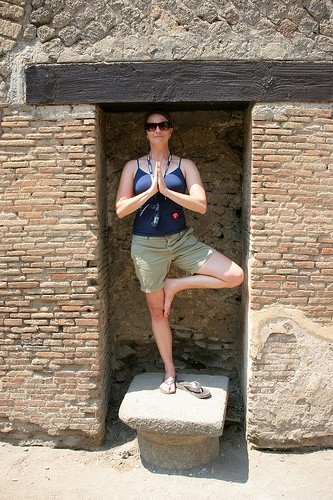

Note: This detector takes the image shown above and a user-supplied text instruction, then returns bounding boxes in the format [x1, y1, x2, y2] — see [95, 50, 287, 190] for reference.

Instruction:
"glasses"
[145, 120, 172, 133]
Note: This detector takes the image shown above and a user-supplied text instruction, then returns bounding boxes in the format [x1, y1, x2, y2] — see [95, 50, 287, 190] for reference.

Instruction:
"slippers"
[159, 374, 178, 395]
[176, 379, 211, 399]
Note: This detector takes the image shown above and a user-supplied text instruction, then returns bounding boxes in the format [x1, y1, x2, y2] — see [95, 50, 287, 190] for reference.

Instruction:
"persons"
[115, 108, 245, 394]
